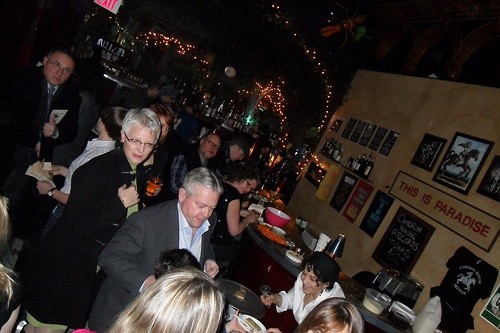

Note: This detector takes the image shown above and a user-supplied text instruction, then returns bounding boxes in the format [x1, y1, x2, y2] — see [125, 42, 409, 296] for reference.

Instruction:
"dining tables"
[232, 215, 413, 333]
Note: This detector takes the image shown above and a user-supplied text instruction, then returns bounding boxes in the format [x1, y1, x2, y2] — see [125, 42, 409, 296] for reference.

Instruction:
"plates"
[388, 301, 416, 326]
[286, 250, 304, 263]
[237, 314, 266, 332]
[214, 278, 266, 319]
[273, 226, 286, 234]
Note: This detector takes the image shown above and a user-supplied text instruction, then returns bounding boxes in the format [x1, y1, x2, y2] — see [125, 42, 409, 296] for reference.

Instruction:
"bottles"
[324, 137, 376, 177]
[412, 296, 442, 333]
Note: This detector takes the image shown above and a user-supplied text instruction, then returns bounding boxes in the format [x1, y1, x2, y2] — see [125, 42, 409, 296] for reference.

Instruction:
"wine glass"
[260, 284, 271, 301]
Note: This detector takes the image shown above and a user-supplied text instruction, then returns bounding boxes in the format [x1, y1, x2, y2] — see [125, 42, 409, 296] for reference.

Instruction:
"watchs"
[47, 188, 57, 197]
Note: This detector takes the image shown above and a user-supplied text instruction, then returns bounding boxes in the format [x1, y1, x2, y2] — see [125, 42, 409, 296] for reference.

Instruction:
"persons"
[85, 167, 225, 333]
[261, 252, 365, 333]
[70, 249, 248, 333]
[0, 50, 82, 241]
[15, 108, 164, 333]
[169, 128, 259, 237]
[36, 102, 176, 245]
[0, 197, 19, 333]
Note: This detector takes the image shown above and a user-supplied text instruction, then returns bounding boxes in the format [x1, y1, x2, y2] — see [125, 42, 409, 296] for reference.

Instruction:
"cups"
[326, 234, 346, 257]
[231, 318, 251, 333]
[145, 172, 163, 196]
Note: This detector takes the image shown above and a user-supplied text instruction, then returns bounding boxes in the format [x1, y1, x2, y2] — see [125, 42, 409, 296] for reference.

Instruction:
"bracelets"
[53, 127, 57, 136]
[273, 293, 280, 304]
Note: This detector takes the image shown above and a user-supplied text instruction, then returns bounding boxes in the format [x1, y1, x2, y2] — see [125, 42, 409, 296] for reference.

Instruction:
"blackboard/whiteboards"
[371, 205, 436, 277]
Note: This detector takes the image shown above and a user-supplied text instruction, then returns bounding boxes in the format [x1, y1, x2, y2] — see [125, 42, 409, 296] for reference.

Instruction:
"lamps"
[224, 51, 242, 78]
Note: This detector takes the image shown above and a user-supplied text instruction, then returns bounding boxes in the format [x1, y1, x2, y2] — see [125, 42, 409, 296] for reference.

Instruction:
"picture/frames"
[410, 132, 448, 173]
[476, 155, 500, 202]
[432, 130, 494, 197]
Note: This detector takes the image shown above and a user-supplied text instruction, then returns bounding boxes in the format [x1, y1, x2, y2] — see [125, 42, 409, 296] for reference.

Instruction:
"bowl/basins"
[266, 206, 291, 227]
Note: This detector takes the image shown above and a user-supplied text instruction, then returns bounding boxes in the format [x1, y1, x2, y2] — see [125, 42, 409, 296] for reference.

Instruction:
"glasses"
[47, 56, 73, 75]
[245, 178, 255, 192]
[124, 129, 158, 148]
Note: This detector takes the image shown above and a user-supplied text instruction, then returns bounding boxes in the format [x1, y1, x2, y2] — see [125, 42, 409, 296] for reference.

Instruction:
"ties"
[46, 86, 55, 116]
